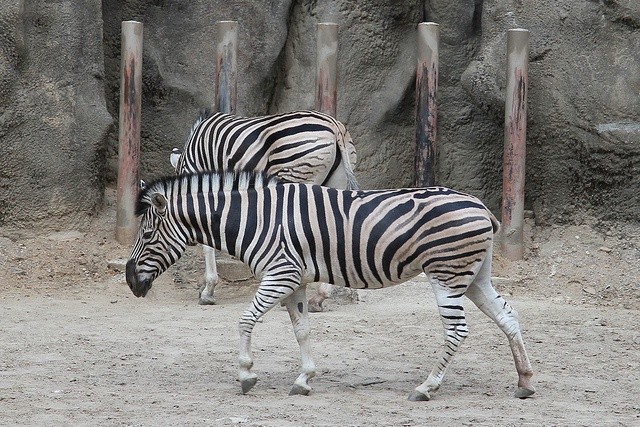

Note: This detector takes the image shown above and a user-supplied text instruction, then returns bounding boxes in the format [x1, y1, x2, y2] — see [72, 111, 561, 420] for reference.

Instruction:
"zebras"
[170, 106, 359, 312]
[125, 172, 537, 402]
